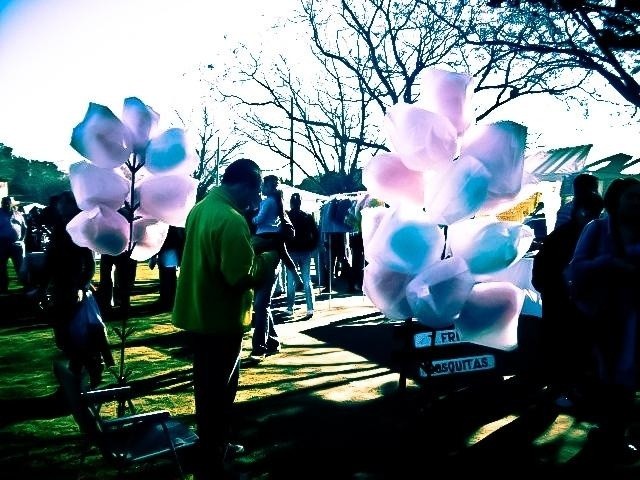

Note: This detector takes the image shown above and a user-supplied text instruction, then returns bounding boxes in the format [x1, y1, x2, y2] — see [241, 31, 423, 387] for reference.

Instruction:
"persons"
[170, 156, 285, 461]
[245, 173, 365, 366]
[523, 170, 639, 480]
[147, 225, 181, 314]
[0, 195, 144, 319]
[30, 188, 118, 404]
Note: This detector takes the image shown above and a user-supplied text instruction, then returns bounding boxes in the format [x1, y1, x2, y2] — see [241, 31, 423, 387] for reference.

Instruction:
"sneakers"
[250, 345, 281, 360]
[284, 309, 293, 315]
[196, 444, 247, 479]
[306, 310, 313, 318]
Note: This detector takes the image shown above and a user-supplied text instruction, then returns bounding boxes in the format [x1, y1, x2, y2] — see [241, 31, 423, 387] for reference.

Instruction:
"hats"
[290, 193, 300, 204]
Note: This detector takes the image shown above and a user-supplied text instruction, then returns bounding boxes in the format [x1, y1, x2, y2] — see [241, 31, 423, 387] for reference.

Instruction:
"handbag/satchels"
[268, 190, 295, 239]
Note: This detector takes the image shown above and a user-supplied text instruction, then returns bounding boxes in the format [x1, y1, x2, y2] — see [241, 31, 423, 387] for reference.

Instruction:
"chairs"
[52, 361, 199, 479]
